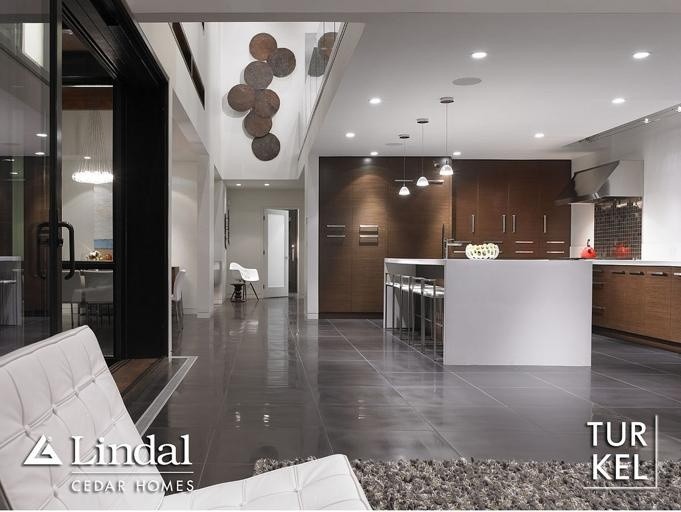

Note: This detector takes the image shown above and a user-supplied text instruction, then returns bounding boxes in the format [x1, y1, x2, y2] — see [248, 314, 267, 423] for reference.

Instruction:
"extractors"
[555, 157, 643, 205]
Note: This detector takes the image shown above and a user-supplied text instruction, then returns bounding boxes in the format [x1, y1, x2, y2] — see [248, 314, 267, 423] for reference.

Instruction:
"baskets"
[465, 243, 500, 259]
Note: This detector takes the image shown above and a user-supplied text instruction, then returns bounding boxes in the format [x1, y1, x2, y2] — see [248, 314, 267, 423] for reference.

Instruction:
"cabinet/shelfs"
[389, 157, 453, 259]
[593, 265, 607, 338]
[383, 257, 594, 367]
[318, 157, 389, 318]
[606, 266, 645, 345]
[452, 160, 479, 259]
[644, 266, 681, 353]
[538, 160, 572, 259]
[478, 161, 539, 259]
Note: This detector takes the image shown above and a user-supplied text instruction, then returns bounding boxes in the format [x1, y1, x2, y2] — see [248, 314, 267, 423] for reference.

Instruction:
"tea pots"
[581, 239, 597, 258]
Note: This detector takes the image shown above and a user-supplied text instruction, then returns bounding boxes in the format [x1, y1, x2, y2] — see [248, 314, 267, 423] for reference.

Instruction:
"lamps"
[69, 110, 113, 185]
[439, 96, 455, 176]
[416, 119, 431, 187]
[398, 134, 411, 197]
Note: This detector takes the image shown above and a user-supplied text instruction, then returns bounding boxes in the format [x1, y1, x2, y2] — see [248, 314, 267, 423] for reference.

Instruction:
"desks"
[61, 260, 113, 270]
[1, 256, 25, 326]
[230, 284, 244, 299]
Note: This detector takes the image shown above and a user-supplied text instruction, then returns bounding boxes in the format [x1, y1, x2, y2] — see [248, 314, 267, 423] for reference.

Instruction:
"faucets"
[443, 238, 463, 259]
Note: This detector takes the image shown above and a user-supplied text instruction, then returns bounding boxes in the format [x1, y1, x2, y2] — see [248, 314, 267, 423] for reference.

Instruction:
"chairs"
[62, 269, 113, 329]
[1, 325, 372, 510]
[384, 271, 445, 360]
[229, 261, 260, 302]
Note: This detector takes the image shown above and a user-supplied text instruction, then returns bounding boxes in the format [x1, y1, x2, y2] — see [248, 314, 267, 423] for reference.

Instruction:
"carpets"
[252, 456, 681, 510]
[104, 355, 199, 437]
